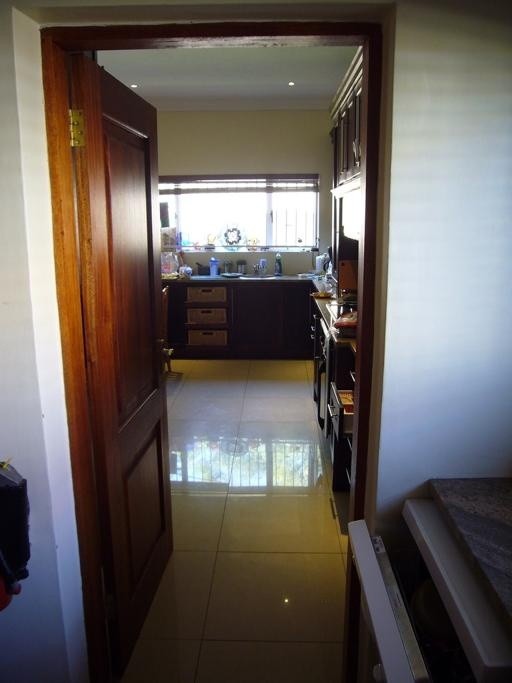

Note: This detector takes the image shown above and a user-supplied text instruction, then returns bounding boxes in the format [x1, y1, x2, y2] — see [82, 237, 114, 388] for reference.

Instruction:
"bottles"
[274, 252, 282, 276]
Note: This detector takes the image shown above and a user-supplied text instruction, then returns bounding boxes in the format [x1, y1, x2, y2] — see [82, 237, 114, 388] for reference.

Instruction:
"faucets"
[253, 264, 259, 271]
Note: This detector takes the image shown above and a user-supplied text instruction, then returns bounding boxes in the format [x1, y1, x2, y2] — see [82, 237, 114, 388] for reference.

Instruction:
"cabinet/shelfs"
[163, 284, 322, 359]
[326, 44, 366, 532]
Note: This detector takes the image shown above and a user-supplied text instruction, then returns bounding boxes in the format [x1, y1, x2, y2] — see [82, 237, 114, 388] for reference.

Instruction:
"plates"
[222, 273, 243, 278]
[309, 291, 332, 299]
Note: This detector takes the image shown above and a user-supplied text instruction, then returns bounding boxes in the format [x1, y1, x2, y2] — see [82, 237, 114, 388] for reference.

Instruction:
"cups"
[209, 257, 219, 277]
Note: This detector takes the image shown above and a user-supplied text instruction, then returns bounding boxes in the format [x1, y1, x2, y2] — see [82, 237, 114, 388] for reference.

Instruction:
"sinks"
[239, 274, 275, 280]
